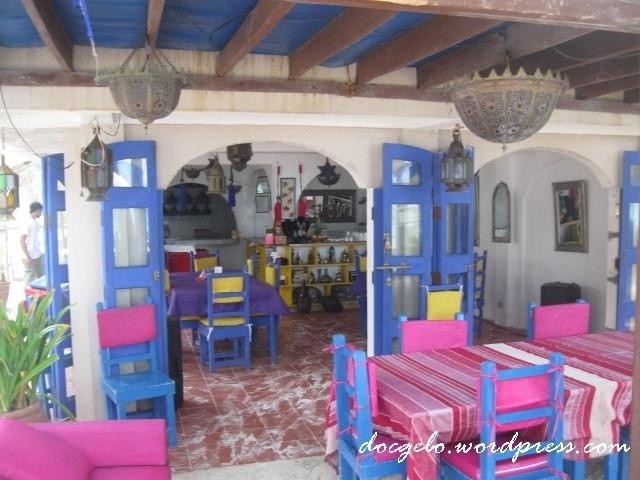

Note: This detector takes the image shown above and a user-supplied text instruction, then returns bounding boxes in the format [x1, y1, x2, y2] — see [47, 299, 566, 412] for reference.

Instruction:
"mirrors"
[300, 189, 356, 223]
[490, 179, 512, 243]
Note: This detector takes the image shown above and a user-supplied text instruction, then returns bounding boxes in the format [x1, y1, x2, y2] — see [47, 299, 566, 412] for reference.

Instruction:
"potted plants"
[307, 215, 328, 241]
[0, 290, 77, 424]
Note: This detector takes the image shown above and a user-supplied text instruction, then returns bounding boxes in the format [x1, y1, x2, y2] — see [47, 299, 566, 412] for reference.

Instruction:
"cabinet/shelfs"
[290, 241, 366, 312]
[247, 242, 289, 308]
[162, 195, 215, 217]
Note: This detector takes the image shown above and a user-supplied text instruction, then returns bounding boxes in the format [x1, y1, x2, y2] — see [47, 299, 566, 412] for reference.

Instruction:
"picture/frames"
[552, 179, 589, 253]
[279, 177, 296, 220]
[255, 175, 270, 194]
[255, 196, 270, 214]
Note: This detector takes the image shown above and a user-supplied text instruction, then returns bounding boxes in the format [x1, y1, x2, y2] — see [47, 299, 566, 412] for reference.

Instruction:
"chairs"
[96, 297, 179, 448]
[440, 351, 567, 479]
[528, 296, 590, 341]
[19, 293, 53, 420]
[400, 313, 466, 353]
[420, 274, 466, 322]
[332, 332, 410, 479]
[475, 249, 487, 337]
[168, 248, 289, 373]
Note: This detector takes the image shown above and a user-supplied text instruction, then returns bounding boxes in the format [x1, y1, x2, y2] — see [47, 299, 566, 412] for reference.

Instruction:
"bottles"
[321, 269, 332, 282]
[291, 249, 313, 264]
[329, 247, 336, 262]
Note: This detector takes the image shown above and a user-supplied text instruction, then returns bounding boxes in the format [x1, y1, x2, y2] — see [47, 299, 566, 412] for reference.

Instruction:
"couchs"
[0, 418, 171, 480]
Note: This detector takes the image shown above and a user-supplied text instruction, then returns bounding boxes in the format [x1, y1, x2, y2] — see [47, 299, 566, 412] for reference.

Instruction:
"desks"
[326, 330, 633, 479]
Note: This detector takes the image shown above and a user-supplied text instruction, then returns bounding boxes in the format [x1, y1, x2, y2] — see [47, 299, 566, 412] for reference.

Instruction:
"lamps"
[92, 0, 186, 131]
[445, 27, 570, 156]
[439, 128, 473, 193]
[227, 143, 252, 172]
[316, 157, 340, 187]
[78, 113, 120, 201]
[1, 128, 20, 223]
